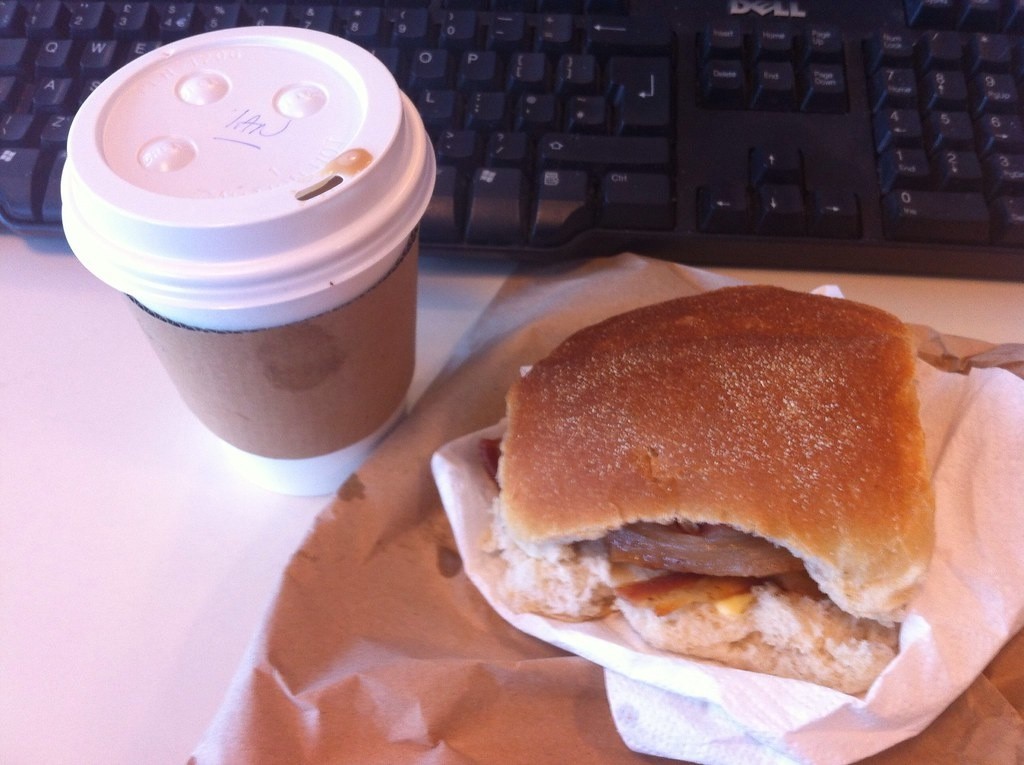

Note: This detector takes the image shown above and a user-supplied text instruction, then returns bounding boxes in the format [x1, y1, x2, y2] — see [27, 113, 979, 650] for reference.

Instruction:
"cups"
[58, 24, 438, 459]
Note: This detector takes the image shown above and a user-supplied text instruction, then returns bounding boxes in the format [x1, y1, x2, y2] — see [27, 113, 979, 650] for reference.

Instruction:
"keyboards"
[0, 0, 1024, 284]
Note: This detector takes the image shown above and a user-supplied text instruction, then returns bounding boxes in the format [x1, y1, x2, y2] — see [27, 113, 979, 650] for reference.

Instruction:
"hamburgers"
[478, 277, 935, 698]
